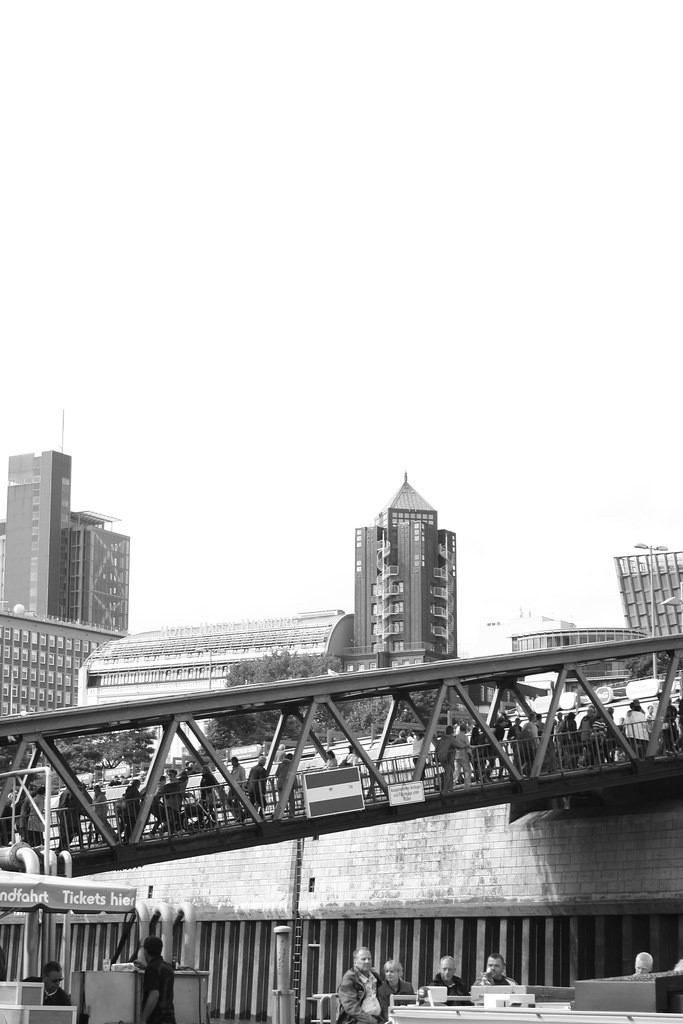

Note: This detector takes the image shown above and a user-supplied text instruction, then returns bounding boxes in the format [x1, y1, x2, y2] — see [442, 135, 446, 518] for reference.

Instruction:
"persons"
[407, 731, 417, 743]
[133, 935, 177, 1024]
[338, 745, 360, 767]
[388, 729, 408, 745]
[335, 946, 384, 1024]
[116, 778, 144, 844]
[430, 954, 474, 1006]
[56, 784, 115, 851]
[471, 953, 519, 986]
[22, 960, 72, 1006]
[413, 731, 429, 782]
[326, 750, 337, 768]
[376, 959, 414, 1024]
[453, 723, 472, 786]
[0, 779, 46, 846]
[150, 753, 294, 838]
[436, 725, 473, 796]
[470, 692, 683, 782]
[631, 952, 653, 975]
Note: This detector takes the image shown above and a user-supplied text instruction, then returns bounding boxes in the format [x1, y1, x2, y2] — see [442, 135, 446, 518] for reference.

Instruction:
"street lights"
[630, 543, 669, 678]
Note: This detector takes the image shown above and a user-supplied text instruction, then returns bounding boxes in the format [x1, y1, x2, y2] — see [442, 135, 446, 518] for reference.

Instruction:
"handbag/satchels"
[339, 759, 351, 768]
[115, 796, 126, 809]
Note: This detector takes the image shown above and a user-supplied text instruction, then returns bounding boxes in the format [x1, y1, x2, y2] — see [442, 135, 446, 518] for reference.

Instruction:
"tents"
[0, 869, 137, 1007]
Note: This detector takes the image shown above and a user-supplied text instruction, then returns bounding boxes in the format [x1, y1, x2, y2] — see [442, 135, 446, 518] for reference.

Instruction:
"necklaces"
[44, 989, 57, 999]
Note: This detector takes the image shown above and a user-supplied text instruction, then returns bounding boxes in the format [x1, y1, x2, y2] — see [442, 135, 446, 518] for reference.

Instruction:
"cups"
[103, 959, 110, 971]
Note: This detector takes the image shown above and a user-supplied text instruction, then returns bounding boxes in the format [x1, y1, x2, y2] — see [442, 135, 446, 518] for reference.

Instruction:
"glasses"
[46, 975, 63, 983]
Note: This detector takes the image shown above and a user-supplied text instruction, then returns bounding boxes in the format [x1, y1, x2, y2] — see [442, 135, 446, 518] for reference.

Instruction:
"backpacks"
[436, 736, 450, 762]
[164, 781, 182, 798]
